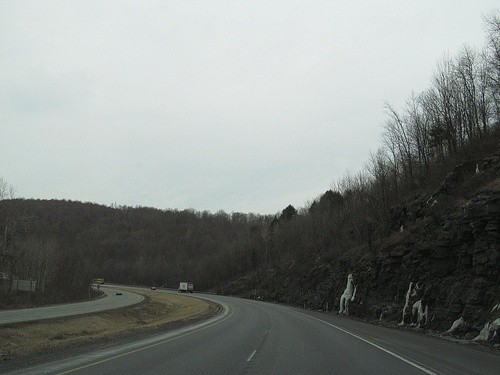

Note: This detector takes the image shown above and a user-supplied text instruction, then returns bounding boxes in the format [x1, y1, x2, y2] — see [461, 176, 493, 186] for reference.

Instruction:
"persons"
[338, 274, 356, 313]
[421, 285, 429, 313]
[410, 282, 422, 302]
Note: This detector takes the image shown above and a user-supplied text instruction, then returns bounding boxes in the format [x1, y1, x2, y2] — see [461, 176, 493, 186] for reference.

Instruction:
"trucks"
[177, 281, 194, 293]
[95, 278, 104, 284]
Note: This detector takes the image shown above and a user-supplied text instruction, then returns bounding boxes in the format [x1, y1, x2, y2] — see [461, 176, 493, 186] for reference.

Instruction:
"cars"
[151, 286, 156, 290]
[115, 289, 122, 295]
